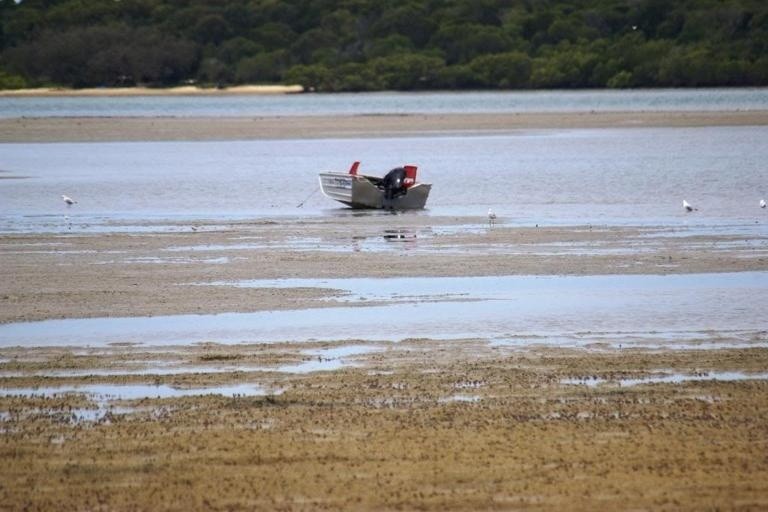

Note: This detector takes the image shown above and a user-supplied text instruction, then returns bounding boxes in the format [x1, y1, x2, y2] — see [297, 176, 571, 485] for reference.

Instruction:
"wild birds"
[61, 194, 77, 213]
[759, 199, 768, 209]
[488, 210, 496, 232]
[682, 199, 698, 215]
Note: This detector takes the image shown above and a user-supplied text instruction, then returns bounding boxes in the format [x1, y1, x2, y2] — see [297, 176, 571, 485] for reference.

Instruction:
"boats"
[317, 171, 431, 211]
